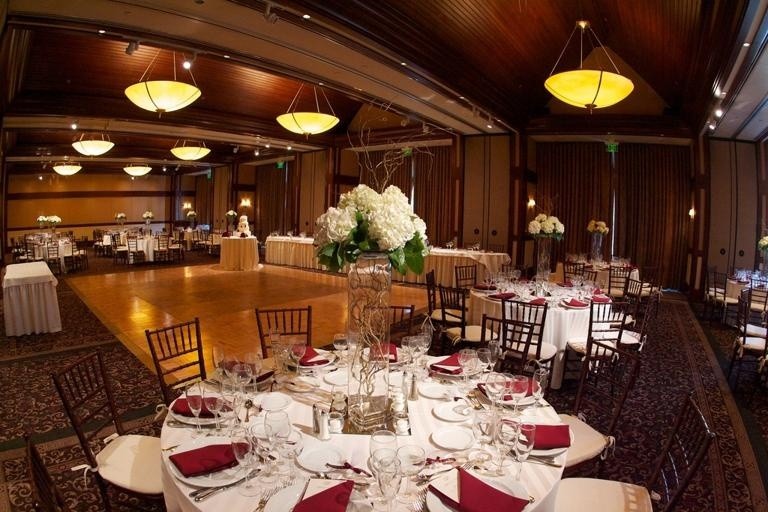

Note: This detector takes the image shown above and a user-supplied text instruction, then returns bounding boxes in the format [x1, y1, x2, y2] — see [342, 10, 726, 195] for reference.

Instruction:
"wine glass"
[446, 242, 453, 249]
[185, 324, 548, 512]
[484, 253, 631, 308]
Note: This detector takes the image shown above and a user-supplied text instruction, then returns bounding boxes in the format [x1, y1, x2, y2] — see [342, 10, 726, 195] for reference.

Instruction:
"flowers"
[46, 216, 62, 224]
[36, 215, 47, 223]
[142, 210, 155, 220]
[527, 214, 566, 242]
[187, 210, 197, 218]
[226, 210, 238, 218]
[586, 219, 609, 237]
[114, 212, 127, 221]
[313, 184, 432, 278]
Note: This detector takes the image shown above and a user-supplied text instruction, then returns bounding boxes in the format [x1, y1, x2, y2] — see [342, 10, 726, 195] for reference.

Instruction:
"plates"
[472, 259, 637, 309]
[167, 346, 574, 512]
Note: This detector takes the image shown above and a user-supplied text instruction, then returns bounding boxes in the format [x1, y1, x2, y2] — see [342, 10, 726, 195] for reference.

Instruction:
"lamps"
[124, 46, 201, 116]
[52, 163, 82, 176]
[170, 136, 211, 161]
[275, 80, 340, 139]
[122, 163, 152, 177]
[72, 132, 114, 156]
[544, 16, 634, 117]
